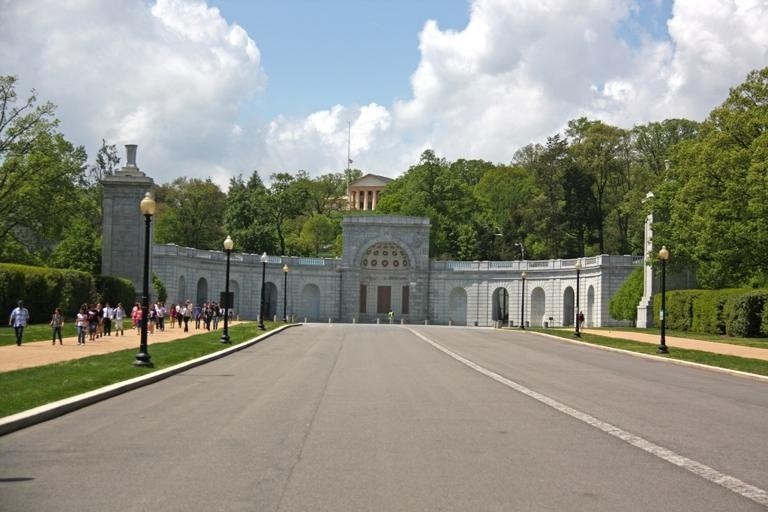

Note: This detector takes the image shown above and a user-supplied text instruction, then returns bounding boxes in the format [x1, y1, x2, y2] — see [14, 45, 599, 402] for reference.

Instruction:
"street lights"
[520, 271, 526, 329]
[515, 242, 524, 260]
[573, 261, 582, 337]
[283, 264, 290, 323]
[657, 245, 670, 354]
[256, 252, 269, 330]
[219, 236, 234, 344]
[131, 192, 156, 367]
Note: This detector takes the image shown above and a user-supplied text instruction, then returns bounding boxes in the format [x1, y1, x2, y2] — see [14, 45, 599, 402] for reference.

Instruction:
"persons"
[228, 305, 234, 321]
[8, 300, 29, 346]
[75, 302, 127, 346]
[49, 308, 64, 345]
[578, 311, 584, 327]
[385, 309, 395, 324]
[130, 299, 225, 336]
